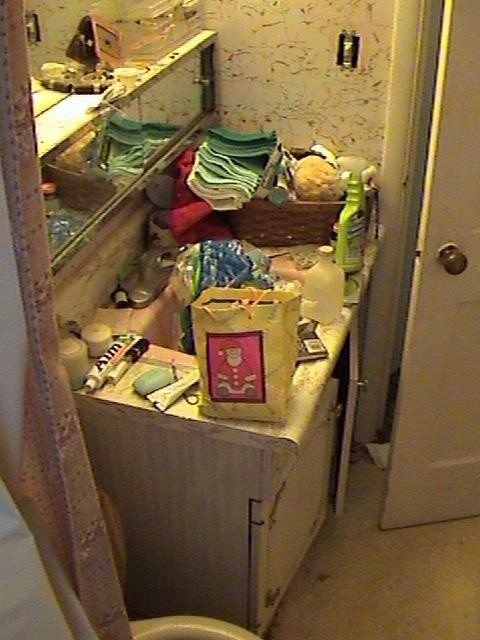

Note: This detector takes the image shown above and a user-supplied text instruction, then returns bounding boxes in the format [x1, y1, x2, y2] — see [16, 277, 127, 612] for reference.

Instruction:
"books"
[297, 328, 329, 364]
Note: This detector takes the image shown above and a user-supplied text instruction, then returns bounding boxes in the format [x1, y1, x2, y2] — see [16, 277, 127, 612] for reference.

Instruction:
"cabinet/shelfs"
[71, 243, 379, 638]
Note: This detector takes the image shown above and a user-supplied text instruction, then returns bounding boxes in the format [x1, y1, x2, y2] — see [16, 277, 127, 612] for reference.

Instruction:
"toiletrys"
[85, 332, 143, 392]
[114, 283, 129, 309]
[80, 323, 113, 359]
[58, 339, 90, 390]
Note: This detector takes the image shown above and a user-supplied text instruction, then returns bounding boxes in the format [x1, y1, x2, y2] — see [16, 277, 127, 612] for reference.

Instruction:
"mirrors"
[23, 1, 207, 275]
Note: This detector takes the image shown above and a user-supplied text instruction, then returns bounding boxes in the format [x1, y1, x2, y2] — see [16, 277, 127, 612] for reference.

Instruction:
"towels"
[96, 116, 277, 243]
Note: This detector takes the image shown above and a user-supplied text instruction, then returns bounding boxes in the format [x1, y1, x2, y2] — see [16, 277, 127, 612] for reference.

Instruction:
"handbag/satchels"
[194, 287, 303, 422]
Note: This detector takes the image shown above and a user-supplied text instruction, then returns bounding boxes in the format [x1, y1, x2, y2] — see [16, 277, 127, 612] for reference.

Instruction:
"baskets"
[227, 197, 373, 248]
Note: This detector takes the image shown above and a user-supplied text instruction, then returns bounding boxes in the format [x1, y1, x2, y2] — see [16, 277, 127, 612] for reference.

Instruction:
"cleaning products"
[337, 156, 370, 273]
